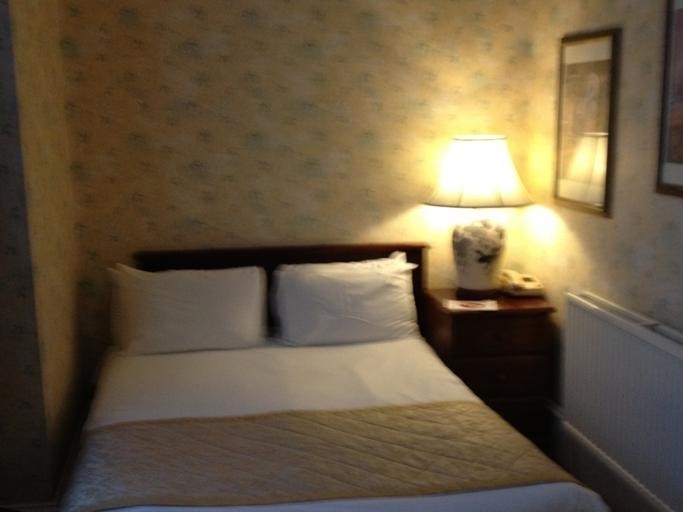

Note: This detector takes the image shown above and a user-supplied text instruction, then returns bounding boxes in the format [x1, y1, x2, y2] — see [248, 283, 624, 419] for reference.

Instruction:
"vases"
[419, 134, 536, 296]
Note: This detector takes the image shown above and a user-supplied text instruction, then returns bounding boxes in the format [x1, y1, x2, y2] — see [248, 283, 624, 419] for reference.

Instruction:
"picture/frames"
[652, 1, 683, 197]
[552, 25, 622, 218]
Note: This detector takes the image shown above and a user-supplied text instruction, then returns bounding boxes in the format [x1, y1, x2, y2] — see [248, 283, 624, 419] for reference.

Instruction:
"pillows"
[105, 263, 269, 357]
[270, 250, 422, 343]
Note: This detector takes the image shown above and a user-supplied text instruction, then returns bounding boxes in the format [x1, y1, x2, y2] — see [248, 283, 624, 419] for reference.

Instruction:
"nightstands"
[422, 287, 557, 404]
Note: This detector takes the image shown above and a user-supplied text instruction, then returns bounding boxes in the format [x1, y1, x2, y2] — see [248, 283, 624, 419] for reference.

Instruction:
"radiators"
[551, 289, 681, 512]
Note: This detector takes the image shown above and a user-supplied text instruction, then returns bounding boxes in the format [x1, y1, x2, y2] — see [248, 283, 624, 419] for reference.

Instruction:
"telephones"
[500, 270, 544, 296]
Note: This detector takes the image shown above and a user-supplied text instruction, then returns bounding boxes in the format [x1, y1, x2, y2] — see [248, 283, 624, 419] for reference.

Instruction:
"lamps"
[567, 132, 607, 206]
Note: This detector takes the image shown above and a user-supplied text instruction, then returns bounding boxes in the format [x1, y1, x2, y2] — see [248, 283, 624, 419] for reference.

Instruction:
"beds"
[54, 242, 608, 511]
